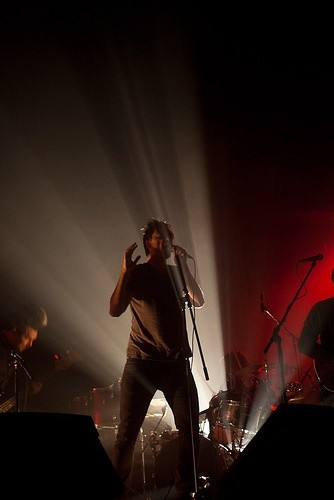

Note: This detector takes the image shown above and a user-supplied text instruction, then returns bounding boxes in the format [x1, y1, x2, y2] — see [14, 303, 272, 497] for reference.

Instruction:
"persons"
[298, 269, 334, 406]
[109, 221, 205, 500]
[0, 301, 47, 413]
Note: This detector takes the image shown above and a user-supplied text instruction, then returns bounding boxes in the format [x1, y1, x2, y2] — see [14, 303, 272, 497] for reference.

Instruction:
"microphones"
[261, 291, 265, 313]
[167, 244, 193, 259]
[300, 253, 324, 263]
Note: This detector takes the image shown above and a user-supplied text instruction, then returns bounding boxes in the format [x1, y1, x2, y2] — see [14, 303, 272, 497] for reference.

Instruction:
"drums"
[152, 434, 234, 500]
[208, 390, 251, 444]
[91, 387, 120, 429]
[213, 399, 263, 434]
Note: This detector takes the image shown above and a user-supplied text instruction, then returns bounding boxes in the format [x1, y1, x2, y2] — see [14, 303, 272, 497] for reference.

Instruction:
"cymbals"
[236, 361, 294, 379]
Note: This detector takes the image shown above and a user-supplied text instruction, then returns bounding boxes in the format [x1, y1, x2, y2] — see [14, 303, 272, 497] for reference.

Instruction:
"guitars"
[0, 349, 81, 413]
[313, 355, 334, 392]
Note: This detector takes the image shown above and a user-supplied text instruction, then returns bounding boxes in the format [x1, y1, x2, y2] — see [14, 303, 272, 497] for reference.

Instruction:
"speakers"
[204, 402, 334, 500]
[0, 412, 127, 500]
